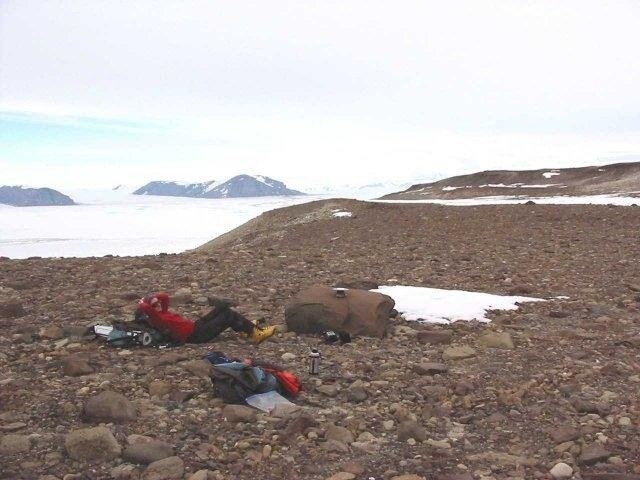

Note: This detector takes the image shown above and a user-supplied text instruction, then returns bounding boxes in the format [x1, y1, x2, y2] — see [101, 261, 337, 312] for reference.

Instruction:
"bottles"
[309, 348, 319, 376]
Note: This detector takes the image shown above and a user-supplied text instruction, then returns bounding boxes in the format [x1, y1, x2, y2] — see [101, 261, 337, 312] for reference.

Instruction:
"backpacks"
[106, 319, 163, 347]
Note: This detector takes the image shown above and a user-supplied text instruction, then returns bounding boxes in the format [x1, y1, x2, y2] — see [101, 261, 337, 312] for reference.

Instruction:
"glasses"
[152, 300, 163, 307]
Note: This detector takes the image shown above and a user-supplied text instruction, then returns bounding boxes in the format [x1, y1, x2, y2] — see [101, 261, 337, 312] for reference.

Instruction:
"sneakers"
[248, 326, 275, 345]
[238, 320, 259, 339]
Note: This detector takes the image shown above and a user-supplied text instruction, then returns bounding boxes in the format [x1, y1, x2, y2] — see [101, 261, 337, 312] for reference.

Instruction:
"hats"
[144, 294, 158, 304]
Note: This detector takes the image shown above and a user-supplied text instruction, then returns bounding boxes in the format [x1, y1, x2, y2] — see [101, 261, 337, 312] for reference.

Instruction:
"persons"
[137, 291, 278, 345]
